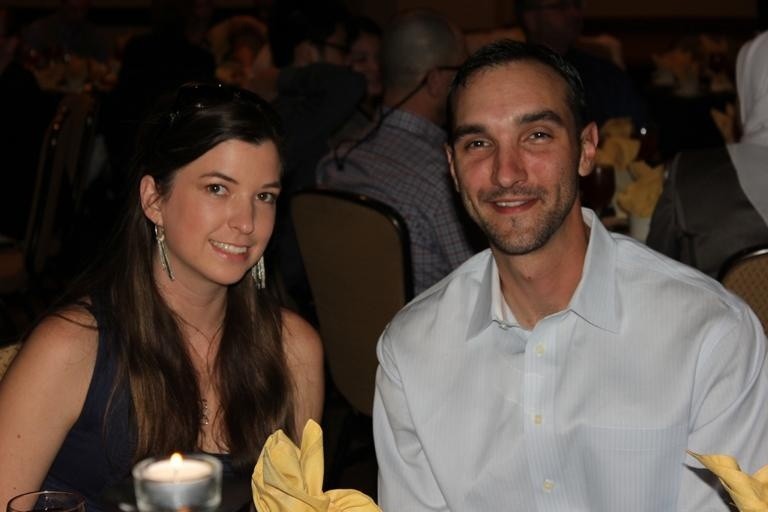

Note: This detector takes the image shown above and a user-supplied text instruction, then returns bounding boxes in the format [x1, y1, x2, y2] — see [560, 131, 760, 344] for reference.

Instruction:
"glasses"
[167, 78, 278, 125]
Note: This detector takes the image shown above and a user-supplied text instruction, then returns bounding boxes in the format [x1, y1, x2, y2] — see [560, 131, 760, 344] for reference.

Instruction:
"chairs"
[718, 244, 768, 336]
[285, 190, 416, 420]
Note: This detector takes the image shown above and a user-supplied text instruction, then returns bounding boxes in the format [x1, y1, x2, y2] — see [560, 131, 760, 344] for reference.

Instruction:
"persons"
[368, 38, 768, 512]
[1, 76, 328, 512]
[1, 1, 767, 298]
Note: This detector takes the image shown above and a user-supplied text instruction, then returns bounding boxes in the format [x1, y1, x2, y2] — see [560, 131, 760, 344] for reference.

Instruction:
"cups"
[635, 124, 667, 170]
[629, 216, 656, 242]
[5, 489, 89, 512]
[589, 163, 617, 213]
